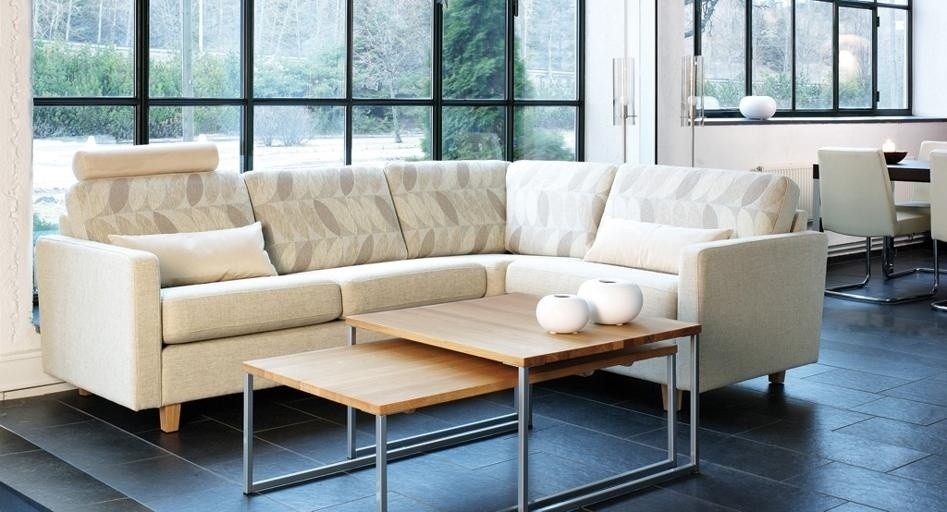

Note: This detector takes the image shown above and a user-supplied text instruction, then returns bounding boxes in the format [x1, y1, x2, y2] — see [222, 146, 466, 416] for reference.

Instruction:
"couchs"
[30, 136, 829, 437]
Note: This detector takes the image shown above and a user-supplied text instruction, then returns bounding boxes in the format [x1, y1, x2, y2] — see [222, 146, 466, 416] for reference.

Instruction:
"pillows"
[580, 216, 735, 275]
[105, 219, 280, 287]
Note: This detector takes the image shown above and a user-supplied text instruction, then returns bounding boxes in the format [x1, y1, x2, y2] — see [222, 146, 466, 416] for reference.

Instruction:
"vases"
[736, 95, 777, 119]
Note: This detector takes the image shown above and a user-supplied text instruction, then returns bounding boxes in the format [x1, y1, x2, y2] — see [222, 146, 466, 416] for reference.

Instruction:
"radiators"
[747, 160, 820, 226]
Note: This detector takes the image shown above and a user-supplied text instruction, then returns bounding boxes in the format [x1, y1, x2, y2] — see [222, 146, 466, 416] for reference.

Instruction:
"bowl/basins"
[882, 152, 907, 165]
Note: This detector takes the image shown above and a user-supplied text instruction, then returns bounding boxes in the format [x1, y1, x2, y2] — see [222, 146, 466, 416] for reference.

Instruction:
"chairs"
[925, 144, 947, 316]
[816, 143, 939, 308]
[911, 140, 946, 204]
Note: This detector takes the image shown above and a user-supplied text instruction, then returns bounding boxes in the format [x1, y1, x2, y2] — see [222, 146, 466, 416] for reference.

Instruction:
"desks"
[812, 155, 946, 283]
[335, 283, 710, 512]
[233, 340, 680, 511]
